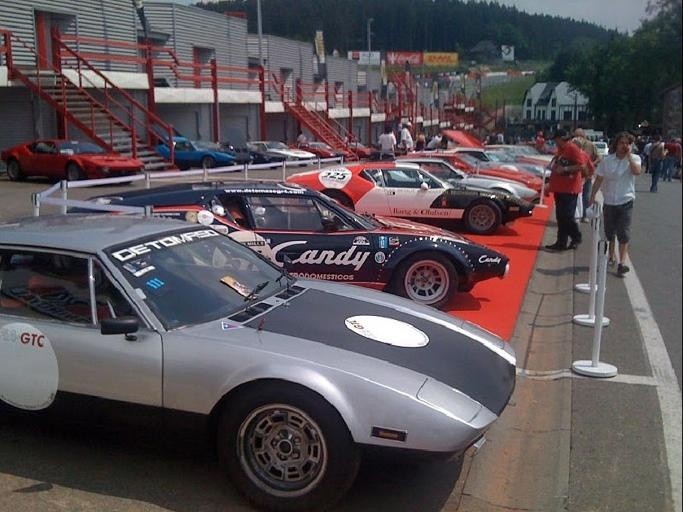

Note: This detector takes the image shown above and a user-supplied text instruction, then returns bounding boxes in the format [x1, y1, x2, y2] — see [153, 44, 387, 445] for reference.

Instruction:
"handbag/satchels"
[547, 156, 571, 176]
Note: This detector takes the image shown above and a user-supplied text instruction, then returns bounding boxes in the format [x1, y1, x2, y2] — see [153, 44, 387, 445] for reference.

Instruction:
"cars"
[592, 140, 611, 157]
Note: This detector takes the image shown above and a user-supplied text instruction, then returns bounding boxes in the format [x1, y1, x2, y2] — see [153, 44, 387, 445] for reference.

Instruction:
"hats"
[548, 129, 566, 139]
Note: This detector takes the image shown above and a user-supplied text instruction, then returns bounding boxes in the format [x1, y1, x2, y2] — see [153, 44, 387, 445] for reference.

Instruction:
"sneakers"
[546, 242, 568, 250]
[608, 255, 616, 267]
[617, 263, 629, 276]
[568, 237, 582, 248]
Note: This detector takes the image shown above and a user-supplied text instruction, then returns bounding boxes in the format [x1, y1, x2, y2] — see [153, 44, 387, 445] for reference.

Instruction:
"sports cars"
[283, 140, 553, 231]
[221, 132, 382, 166]
[55, 181, 510, 311]
[1, 213, 521, 512]
[2, 134, 142, 186]
[139, 128, 255, 176]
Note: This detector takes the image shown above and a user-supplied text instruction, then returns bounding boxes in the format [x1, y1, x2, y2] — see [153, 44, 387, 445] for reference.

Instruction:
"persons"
[296, 130, 306, 149]
[378, 121, 448, 160]
[534, 128, 683, 275]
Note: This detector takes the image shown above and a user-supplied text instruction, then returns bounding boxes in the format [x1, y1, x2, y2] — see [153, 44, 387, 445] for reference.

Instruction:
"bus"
[581, 130, 605, 142]
